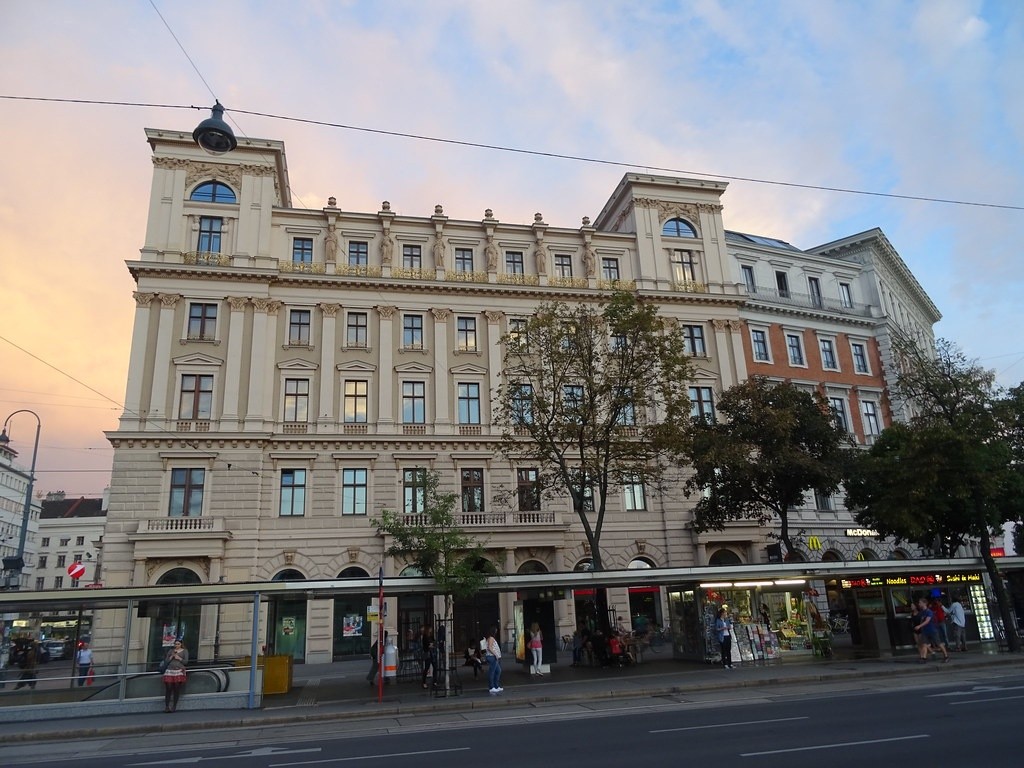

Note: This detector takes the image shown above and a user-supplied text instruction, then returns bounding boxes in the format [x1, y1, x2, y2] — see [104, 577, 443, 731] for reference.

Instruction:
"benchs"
[456, 660, 489, 671]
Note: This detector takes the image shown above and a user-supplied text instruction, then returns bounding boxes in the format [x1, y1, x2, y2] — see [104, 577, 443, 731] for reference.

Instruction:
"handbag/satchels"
[527, 641, 532, 649]
[159, 660, 166, 674]
[86, 670, 95, 686]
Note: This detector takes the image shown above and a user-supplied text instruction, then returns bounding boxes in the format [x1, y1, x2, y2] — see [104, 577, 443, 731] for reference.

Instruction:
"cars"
[79, 634, 91, 649]
[44, 639, 73, 661]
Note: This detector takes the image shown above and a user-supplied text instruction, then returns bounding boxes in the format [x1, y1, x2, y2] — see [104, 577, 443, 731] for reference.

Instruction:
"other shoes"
[960, 646, 968, 651]
[918, 657, 928, 664]
[489, 687, 503, 692]
[421, 683, 429, 689]
[951, 647, 961, 652]
[724, 665, 736, 669]
[535, 669, 544, 675]
[930, 653, 936, 661]
[943, 655, 950, 662]
[165, 705, 176, 712]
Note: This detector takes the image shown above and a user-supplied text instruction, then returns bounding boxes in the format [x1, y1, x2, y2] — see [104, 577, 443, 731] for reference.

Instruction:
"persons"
[714, 608, 736, 670]
[420, 624, 439, 689]
[484, 625, 504, 693]
[569, 616, 628, 670]
[76, 643, 94, 686]
[909, 597, 966, 664]
[366, 631, 391, 689]
[527, 622, 544, 676]
[463, 639, 483, 677]
[162, 639, 189, 713]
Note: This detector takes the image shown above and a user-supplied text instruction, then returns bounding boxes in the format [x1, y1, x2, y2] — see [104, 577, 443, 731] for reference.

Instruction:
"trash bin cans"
[383, 636, 399, 678]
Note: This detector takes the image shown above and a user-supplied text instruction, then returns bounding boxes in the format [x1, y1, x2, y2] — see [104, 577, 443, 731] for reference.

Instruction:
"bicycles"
[650, 624, 674, 655]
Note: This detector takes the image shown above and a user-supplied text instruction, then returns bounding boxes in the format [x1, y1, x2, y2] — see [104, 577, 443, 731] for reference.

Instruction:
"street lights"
[0, 409, 44, 557]
